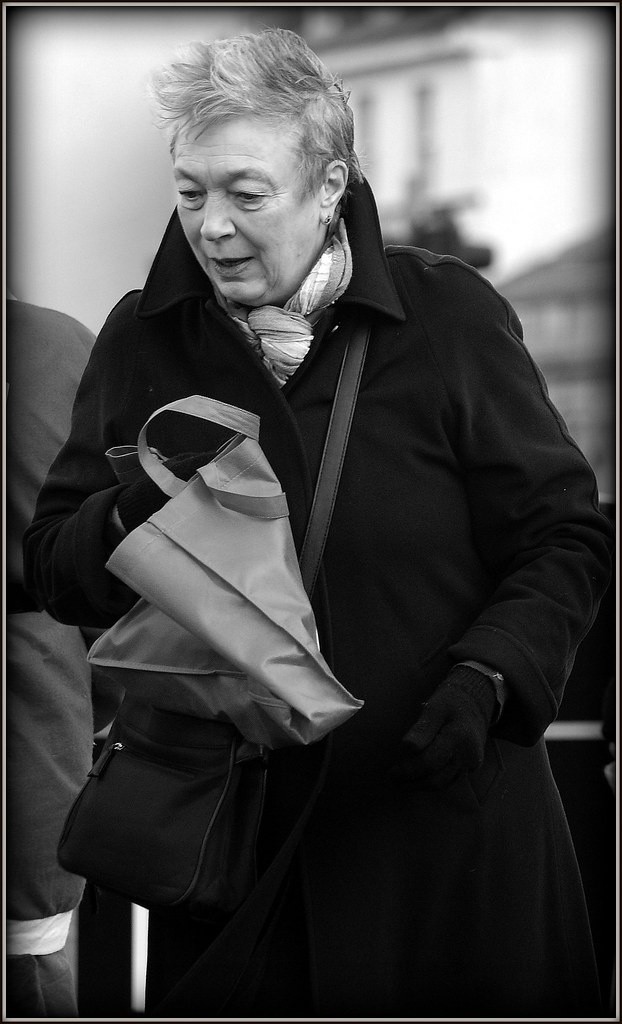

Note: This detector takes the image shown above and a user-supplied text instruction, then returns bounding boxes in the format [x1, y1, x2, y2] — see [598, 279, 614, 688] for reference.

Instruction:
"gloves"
[406, 667, 504, 792]
[116, 441, 218, 535]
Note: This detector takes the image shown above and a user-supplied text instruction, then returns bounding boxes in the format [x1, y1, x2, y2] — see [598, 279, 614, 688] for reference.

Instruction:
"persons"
[18, 25, 609, 1017]
[7, 298, 98, 1018]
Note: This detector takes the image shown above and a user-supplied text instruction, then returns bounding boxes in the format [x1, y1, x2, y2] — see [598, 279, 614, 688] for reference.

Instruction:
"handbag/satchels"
[58, 720, 273, 916]
[84, 394, 364, 749]
